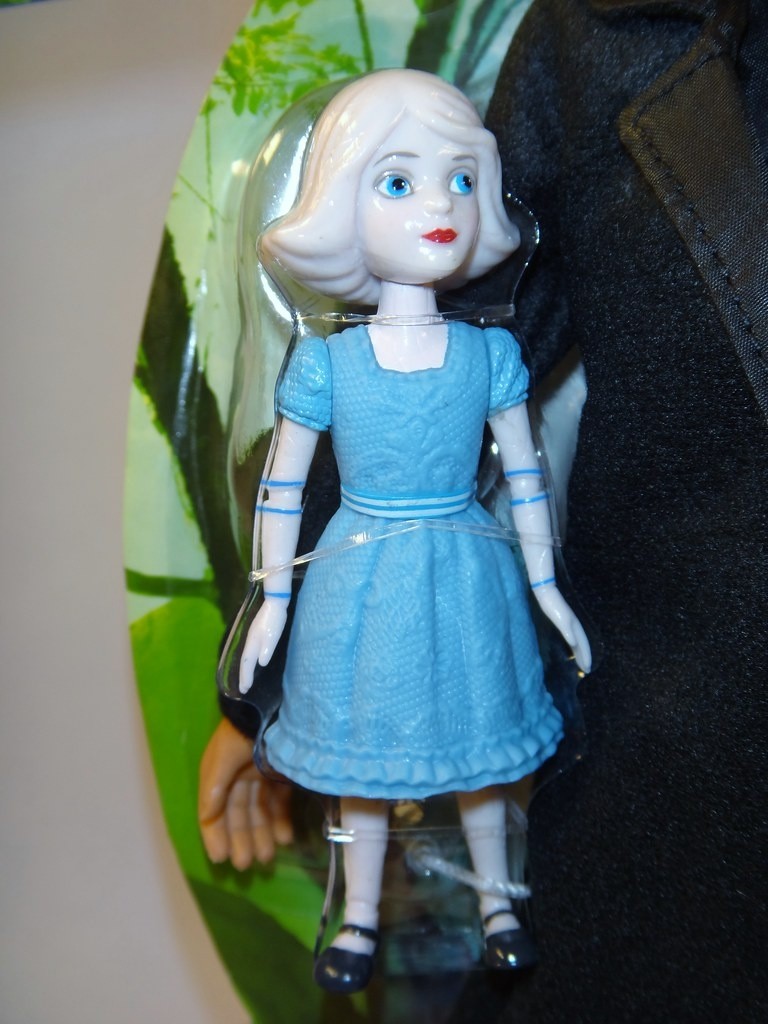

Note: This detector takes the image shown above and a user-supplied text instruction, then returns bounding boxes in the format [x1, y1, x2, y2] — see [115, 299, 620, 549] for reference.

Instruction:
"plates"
[123, 0, 767, 1024]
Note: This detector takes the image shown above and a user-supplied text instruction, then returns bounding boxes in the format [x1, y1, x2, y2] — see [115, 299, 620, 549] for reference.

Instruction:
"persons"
[197, 0, 767, 1024]
